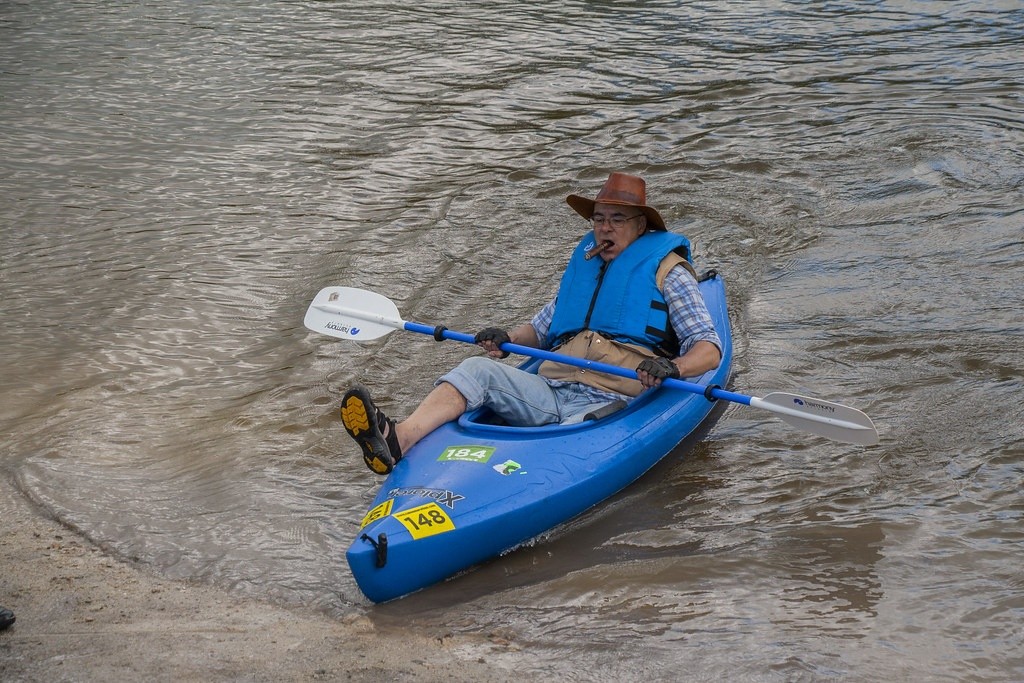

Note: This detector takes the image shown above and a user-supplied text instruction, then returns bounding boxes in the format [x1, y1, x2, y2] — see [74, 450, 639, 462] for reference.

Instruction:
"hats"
[567, 171, 668, 234]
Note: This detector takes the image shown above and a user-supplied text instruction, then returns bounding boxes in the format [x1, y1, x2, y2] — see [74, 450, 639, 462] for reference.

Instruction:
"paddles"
[303, 286, 881, 448]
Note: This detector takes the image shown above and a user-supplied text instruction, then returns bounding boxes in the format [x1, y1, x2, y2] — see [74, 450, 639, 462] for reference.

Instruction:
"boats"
[345, 268, 731, 605]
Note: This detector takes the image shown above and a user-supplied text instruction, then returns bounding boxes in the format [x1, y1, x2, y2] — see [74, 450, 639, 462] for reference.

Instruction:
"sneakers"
[340, 385, 400, 475]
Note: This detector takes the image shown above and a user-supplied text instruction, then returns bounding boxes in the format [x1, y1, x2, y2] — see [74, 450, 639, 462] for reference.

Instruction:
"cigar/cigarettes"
[584, 241, 612, 261]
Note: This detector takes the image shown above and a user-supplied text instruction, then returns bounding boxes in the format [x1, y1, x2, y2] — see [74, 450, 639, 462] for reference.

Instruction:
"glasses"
[586, 213, 646, 228]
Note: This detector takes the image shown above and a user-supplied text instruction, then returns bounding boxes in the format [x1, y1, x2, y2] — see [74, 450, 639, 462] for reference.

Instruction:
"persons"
[340, 172, 724, 475]
